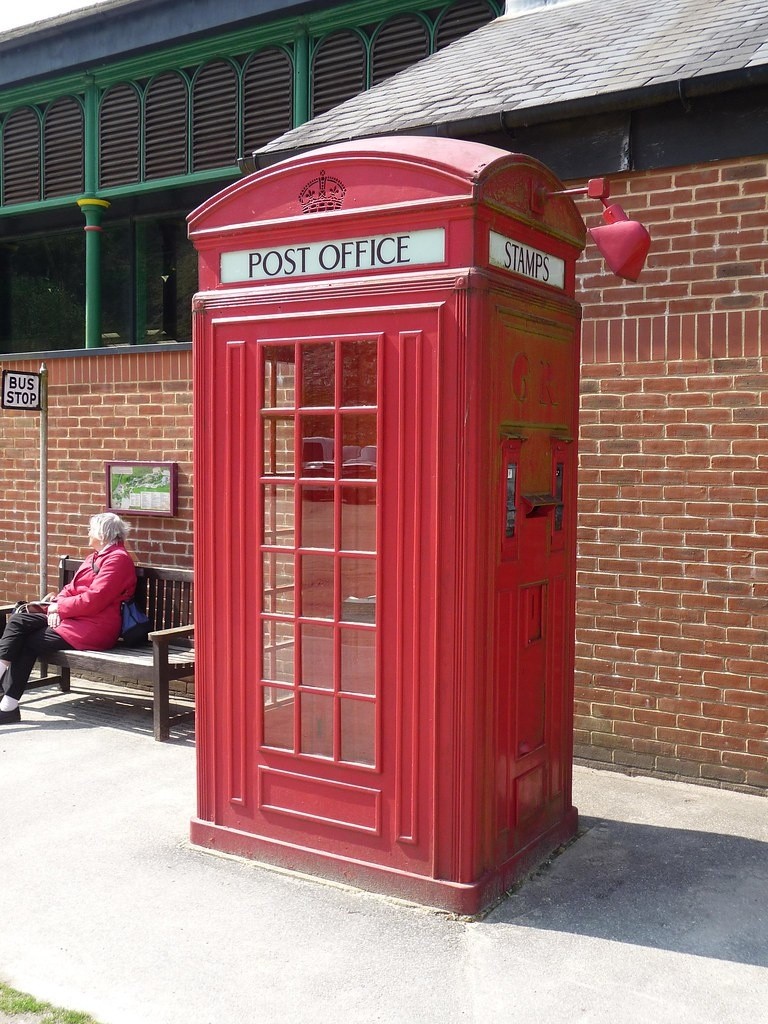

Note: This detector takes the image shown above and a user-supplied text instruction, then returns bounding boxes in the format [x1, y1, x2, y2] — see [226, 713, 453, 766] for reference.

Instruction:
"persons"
[0, 513, 137, 724]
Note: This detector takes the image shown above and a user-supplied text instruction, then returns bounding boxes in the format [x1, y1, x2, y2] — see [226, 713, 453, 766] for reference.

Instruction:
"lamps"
[530, 174, 652, 281]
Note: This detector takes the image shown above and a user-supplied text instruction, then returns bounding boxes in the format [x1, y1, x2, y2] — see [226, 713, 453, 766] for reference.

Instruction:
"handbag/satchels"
[14, 592, 55, 613]
[120, 601, 154, 650]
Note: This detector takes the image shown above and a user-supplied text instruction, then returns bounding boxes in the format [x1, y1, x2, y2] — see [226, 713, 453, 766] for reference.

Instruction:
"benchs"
[0, 555, 195, 741]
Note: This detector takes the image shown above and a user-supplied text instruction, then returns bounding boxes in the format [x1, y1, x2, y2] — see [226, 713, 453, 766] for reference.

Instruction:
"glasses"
[88, 527, 91, 532]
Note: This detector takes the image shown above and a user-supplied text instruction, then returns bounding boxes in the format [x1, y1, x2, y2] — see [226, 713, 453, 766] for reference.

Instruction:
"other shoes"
[0, 670, 7, 687]
[0, 705, 21, 725]
